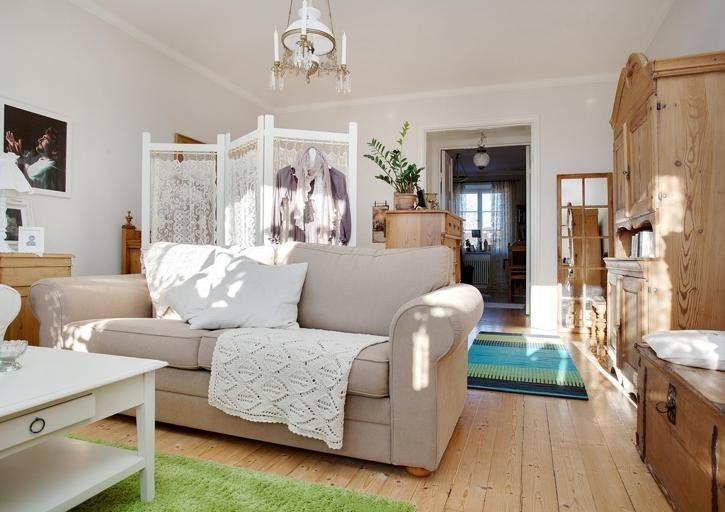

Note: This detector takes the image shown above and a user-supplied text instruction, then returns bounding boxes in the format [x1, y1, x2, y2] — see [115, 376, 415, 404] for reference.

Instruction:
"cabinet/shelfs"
[0, 252, 72, 346]
[385, 209, 466, 283]
[602, 255, 658, 399]
[613, 93, 658, 226]
[631, 342, 725, 512]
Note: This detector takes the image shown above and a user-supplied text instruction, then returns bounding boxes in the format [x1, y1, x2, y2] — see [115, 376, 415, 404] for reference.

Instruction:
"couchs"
[28, 237, 485, 478]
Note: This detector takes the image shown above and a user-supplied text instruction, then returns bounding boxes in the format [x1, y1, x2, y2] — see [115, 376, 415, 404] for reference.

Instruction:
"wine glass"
[0, 340, 28, 373]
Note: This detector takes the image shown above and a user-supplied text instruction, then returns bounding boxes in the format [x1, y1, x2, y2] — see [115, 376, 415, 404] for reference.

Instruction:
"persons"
[4, 125, 65, 190]
[5, 209, 22, 241]
[26, 235, 36, 246]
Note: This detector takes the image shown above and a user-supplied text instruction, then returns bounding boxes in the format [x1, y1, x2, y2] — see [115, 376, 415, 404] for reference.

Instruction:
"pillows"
[159, 247, 240, 324]
[188, 244, 309, 330]
[139, 241, 188, 320]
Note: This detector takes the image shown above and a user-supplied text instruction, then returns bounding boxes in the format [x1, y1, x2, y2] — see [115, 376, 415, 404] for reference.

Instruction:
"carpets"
[467, 331, 588, 400]
[61, 430, 418, 512]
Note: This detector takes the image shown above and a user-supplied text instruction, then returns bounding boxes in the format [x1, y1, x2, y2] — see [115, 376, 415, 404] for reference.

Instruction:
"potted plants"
[362, 120, 425, 210]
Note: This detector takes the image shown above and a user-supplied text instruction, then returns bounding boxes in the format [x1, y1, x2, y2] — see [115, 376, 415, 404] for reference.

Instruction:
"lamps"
[270, 0, 354, 95]
[0, 151, 33, 253]
[472, 130, 491, 169]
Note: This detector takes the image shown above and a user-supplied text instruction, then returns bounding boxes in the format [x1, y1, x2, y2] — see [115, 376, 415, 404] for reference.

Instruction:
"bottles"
[483, 238, 488, 250]
[478, 239, 482, 252]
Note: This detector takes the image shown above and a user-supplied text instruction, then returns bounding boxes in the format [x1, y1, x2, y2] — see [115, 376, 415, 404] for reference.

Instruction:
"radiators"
[464, 252, 491, 285]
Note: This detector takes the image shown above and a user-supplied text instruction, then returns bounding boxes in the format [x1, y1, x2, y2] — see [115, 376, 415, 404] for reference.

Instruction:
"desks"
[504, 259, 526, 303]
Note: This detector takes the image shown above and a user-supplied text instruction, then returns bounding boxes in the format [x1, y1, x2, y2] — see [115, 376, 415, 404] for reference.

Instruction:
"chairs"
[507, 240, 526, 298]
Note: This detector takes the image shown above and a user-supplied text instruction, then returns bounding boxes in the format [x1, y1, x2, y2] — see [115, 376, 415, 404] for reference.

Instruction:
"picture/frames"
[0, 97, 74, 199]
[17, 225, 45, 253]
[6, 200, 26, 244]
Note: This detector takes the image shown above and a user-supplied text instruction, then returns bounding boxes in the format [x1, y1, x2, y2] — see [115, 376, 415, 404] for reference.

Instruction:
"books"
[631, 230, 656, 258]
